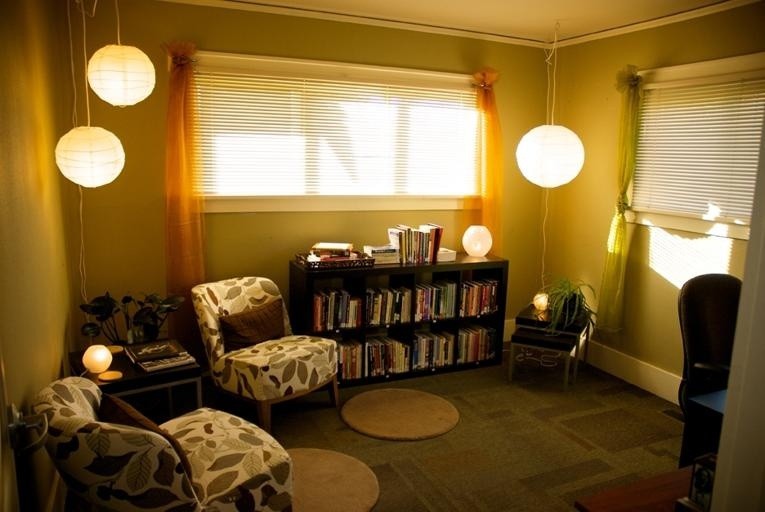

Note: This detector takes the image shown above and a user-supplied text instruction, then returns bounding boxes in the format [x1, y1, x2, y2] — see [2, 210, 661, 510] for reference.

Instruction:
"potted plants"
[540, 270, 627, 337]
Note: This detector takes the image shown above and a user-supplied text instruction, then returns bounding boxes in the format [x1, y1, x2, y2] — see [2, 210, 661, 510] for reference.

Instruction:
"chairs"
[29, 377, 295, 511]
[189, 273, 344, 434]
[675, 270, 742, 413]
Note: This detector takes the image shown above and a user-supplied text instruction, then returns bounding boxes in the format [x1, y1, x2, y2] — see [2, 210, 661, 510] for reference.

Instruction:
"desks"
[679, 385, 729, 467]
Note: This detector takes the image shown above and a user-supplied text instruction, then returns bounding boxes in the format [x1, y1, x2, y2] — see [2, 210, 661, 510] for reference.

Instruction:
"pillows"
[220, 297, 290, 352]
[96, 393, 193, 483]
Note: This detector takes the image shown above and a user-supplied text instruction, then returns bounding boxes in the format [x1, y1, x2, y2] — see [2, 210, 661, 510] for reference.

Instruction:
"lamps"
[48, 1, 158, 191]
[461, 224, 498, 259]
[81, 343, 116, 374]
[515, 30, 586, 190]
[533, 292, 550, 311]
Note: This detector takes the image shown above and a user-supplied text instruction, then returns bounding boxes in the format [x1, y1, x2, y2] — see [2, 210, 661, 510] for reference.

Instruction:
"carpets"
[285, 448, 381, 512]
[340, 388, 461, 443]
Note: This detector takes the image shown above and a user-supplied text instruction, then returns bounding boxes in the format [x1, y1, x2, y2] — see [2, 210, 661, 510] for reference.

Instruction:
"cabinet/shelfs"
[292, 264, 511, 389]
[505, 301, 592, 389]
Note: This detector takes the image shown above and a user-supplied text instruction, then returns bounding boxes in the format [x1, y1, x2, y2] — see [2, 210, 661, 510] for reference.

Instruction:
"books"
[122, 338, 189, 362]
[307, 223, 500, 379]
[137, 353, 197, 371]
